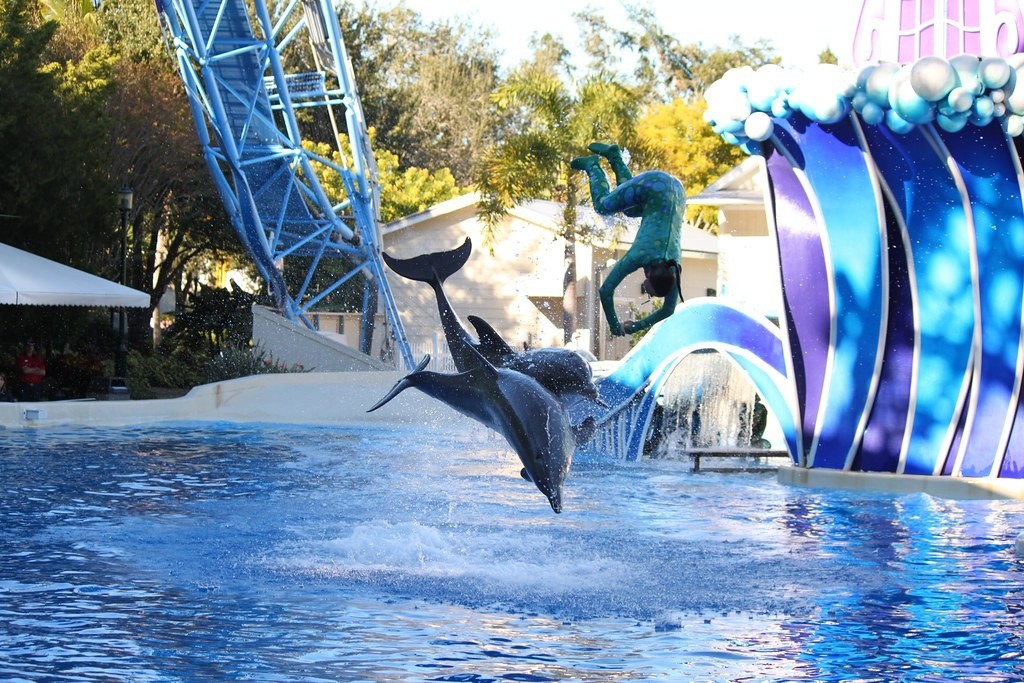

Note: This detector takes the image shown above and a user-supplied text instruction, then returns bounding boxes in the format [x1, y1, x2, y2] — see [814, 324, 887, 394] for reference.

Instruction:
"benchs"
[684, 447, 788, 471]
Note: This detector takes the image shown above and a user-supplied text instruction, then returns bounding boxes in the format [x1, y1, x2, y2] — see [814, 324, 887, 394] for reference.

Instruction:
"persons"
[692, 394, 767, 444]
[16, 339, 46, 401]
[571, 142, 686, 336]
[0, 373, 15, 402]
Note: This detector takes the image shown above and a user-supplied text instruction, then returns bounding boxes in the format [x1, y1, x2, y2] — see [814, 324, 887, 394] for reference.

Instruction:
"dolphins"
[382, 236, 598, 400]
[365, 337, 576, 514]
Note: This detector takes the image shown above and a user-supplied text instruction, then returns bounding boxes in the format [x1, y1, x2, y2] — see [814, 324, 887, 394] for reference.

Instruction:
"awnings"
[0, 241, 152, 309]
